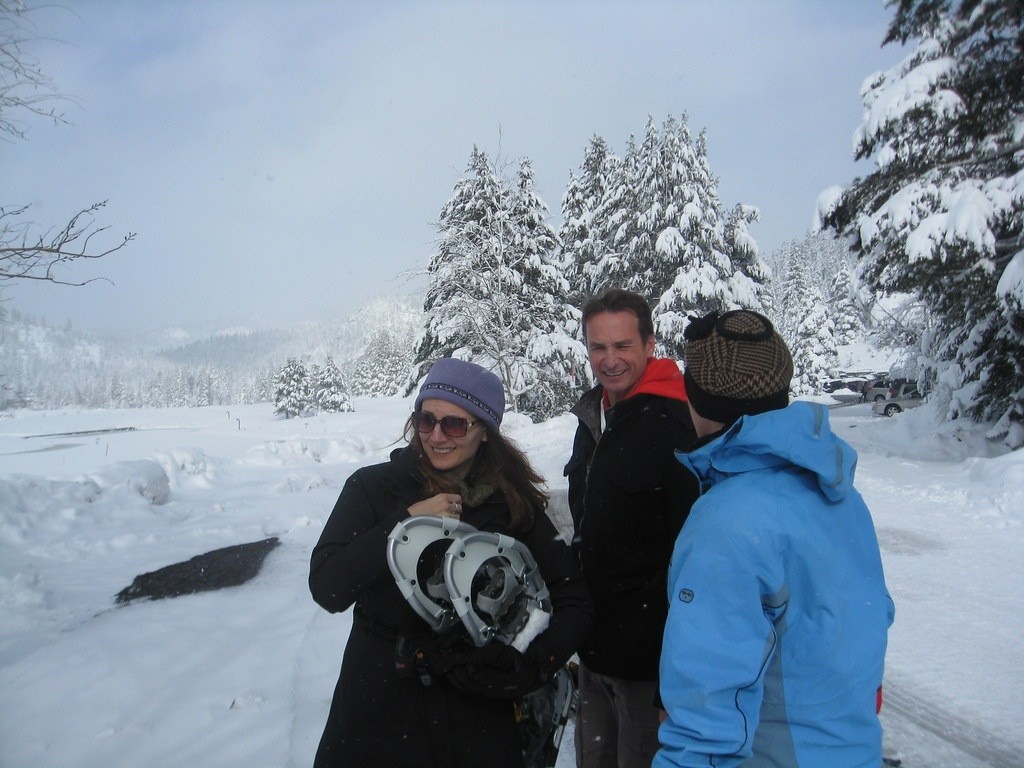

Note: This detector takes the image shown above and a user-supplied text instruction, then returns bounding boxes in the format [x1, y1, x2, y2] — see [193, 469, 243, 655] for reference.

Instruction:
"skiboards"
[387, 516, 579, 768]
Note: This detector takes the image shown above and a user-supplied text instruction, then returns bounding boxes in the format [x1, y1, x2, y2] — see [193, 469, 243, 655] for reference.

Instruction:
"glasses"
[410, 410, 481, 438]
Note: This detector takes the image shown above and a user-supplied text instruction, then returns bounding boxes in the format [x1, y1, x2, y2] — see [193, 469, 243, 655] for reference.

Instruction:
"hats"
[683, 309, 795, 425]
[414, 357, 506, 435]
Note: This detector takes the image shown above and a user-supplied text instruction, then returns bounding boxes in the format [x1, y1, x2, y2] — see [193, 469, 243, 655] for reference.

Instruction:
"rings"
[451, 501, 458, 514]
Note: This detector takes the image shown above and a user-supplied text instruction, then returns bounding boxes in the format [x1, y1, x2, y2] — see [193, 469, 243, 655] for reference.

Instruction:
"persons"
[309, 358, 595, 768]
[649, 309, 895, 768]
[563, 290, 699, 768]
